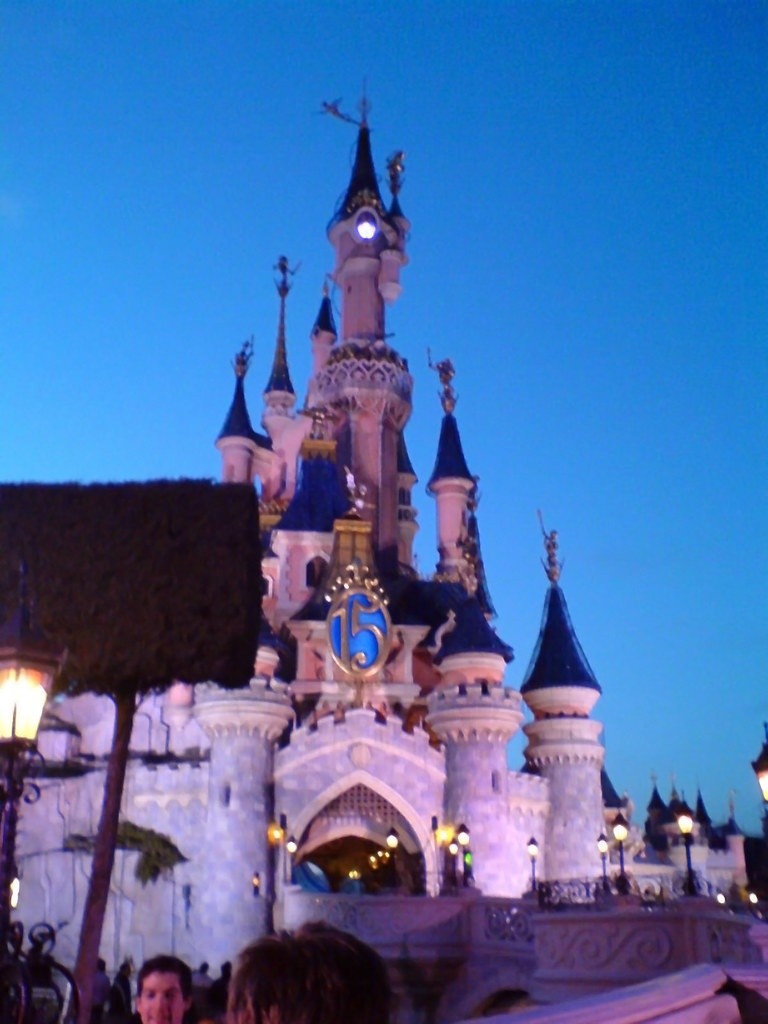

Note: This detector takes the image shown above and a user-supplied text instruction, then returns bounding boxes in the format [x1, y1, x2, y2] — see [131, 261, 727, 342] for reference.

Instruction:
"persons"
[90, 922, 392, 1024]
[538, 880, 762, 918]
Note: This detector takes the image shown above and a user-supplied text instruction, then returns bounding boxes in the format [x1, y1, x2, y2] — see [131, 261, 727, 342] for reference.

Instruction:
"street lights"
[387, 834, 402, 885]
[750, 721, 768, 904]
[526, 836, 539, 890]
[287, 834, 300, 885]
[610, 814, 631, 874]
[0, 558, 72, 969]
[448, 841, 461, 884]
[676, 813, 697, 896]
[595, 835, 608, 880]
[456, 825, 469, 886]
